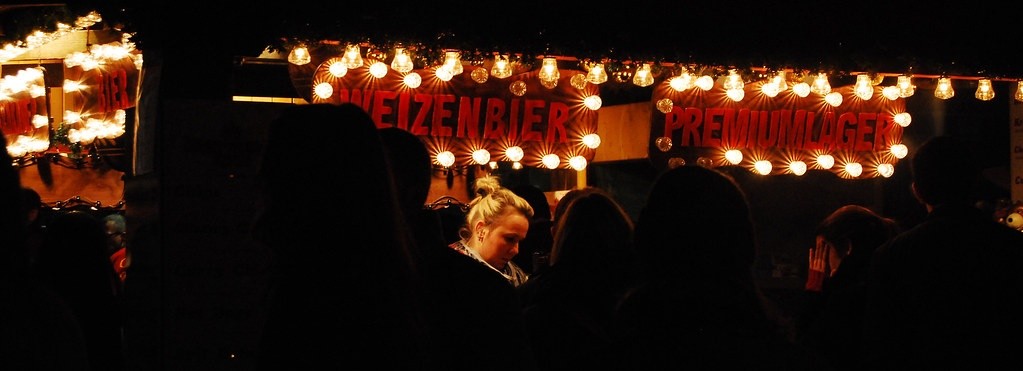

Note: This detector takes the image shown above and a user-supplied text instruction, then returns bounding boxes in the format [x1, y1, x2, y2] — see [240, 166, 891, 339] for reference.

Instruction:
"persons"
[254, 102, 539, 371]
[794, 133, 1022, 371]
[610, 164, 821, 371]
[447, 177, 537, 285]
[501, 167, 640, 371]
[0, 137, 133, 371]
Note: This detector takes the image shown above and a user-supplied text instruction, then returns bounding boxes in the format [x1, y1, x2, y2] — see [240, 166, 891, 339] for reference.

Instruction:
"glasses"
[106, 231, 122, 239]
